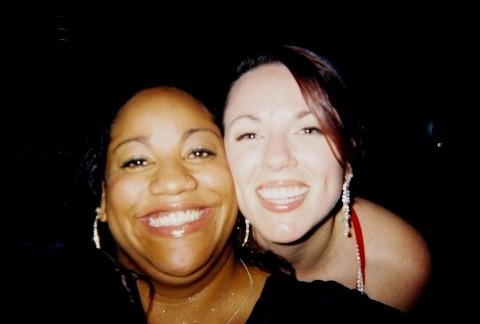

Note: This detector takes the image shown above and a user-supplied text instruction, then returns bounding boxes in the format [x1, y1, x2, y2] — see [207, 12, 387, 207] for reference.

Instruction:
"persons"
[209, 41, 431, 309]
[1, 73, 426, 324]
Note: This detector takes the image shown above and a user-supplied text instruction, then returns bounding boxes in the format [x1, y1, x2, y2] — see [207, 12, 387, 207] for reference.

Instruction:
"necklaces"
[225, 258, 254, 322]
